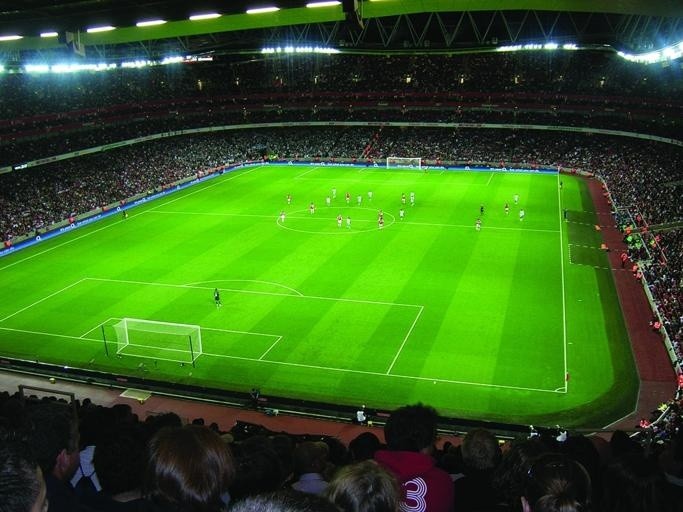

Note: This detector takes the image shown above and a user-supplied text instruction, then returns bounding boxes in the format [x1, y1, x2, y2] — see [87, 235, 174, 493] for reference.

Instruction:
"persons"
[0, 51, 682, 512]
